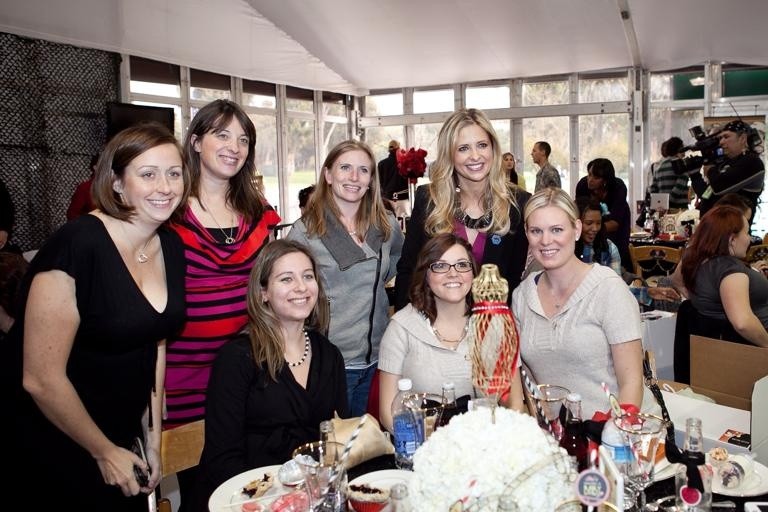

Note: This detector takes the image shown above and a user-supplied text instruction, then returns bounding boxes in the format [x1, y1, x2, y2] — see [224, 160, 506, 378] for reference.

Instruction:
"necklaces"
[284, 329, 310, 366]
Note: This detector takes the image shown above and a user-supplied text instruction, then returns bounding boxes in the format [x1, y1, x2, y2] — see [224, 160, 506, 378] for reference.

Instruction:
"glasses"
[427, 261, 473, 273]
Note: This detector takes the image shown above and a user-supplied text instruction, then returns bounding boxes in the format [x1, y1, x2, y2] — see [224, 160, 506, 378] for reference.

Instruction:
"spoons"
[635, 493, 675, 512]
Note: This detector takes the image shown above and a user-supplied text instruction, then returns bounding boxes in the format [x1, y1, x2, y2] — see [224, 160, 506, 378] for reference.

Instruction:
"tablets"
[650, 193, 670, 210]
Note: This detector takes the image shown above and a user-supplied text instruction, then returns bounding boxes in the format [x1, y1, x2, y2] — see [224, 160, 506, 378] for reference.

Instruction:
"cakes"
[401, 408, 579, 512]
[719, 452, 754, 490]
[344, 484, 390, 503]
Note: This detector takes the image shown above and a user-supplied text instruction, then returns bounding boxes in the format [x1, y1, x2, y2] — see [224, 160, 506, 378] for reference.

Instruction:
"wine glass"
[613, 413, 666, 512]
[471, 377, 512, 425]
[533, 383, 571, 443]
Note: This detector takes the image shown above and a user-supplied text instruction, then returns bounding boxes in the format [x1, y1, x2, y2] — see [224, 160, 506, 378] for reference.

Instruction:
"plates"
[702, 450, 768, 497]
[343, 493, 389, 512]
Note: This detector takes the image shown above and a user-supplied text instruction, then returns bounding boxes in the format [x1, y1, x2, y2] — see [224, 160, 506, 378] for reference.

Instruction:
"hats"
[389, 140, 399, 151]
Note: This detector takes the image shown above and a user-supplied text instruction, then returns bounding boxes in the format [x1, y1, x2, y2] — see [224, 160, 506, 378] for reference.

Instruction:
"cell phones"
[129, 435, 152, 487]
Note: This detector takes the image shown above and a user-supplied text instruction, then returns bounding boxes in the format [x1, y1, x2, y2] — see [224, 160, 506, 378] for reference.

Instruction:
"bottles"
[388, 484, 413, 512]
[319, 421, 347, 495]
[392, 377, 429, 471]
[293, 442, 352, 510]
[644, 213, 651, 232]
[435, 382, 464, 436]
[559, 394, 588, 470]
[675, 417, 713, 511]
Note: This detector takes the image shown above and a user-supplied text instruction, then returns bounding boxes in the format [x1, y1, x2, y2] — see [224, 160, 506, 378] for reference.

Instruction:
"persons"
[287, 138, 407, 418]
[377, 235, 523, 436]
[648, 119, 768, 347]
[502, 141, 680, 304]
[393, 108, 532, 313]
[378, 141, 409, 218]
[164, 98, 281, 428]
[510, 186, 645, 425]
[23, 119, 189, 512]
[1, 150, 104, 250]
[198, 238, 346, 512]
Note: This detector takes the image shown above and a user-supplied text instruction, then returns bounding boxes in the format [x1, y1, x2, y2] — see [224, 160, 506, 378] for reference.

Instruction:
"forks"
[653, 498, 736, 512]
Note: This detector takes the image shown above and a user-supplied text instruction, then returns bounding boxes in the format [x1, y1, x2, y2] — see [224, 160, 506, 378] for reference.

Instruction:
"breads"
[240, 474, 273, 499]
[709, 446, 727, 467]
